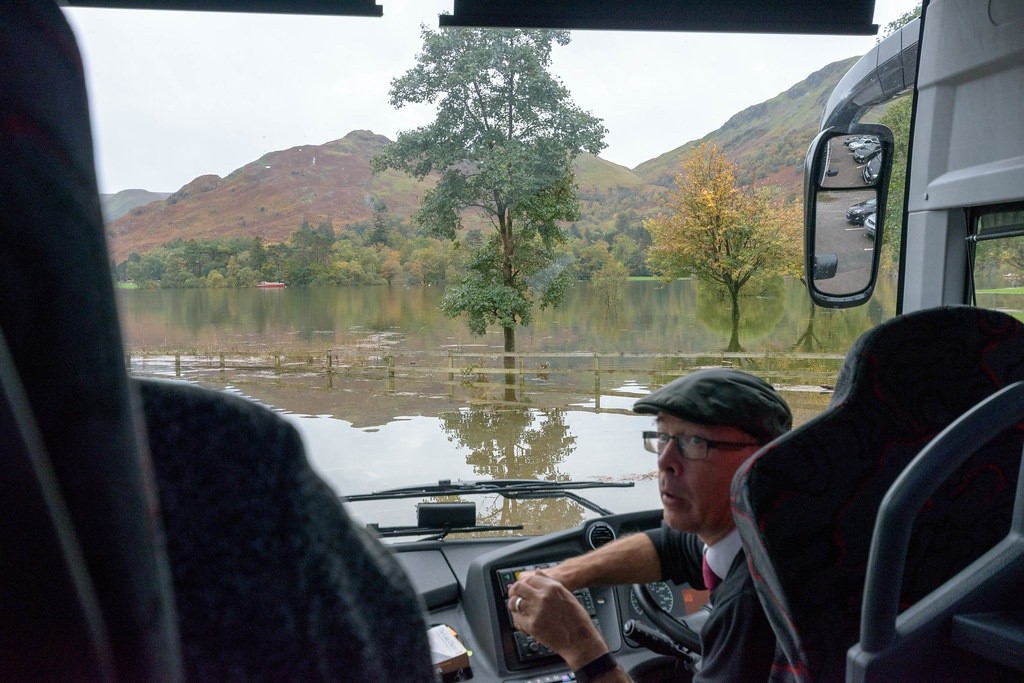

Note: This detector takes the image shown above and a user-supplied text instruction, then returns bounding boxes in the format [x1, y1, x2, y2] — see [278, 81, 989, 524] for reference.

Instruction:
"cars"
[863, 213, 877, 238]
[862, 150, 882, 184]
[842, 134, 881, 165]
[846, 195, 877, 225]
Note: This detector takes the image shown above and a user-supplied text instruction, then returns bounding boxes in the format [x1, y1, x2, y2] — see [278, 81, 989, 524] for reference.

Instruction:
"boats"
[256, 280, 286, 290]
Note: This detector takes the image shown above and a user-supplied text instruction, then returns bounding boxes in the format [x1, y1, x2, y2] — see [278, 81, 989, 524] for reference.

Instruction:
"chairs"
[733, 303, 1024, 683]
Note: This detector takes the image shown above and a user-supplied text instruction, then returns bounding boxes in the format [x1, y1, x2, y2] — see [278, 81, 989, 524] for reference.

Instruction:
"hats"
[632, 368, 792, 445]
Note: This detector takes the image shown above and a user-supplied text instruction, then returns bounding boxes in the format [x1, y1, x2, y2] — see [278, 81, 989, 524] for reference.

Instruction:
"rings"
[516, 597, 522, 612]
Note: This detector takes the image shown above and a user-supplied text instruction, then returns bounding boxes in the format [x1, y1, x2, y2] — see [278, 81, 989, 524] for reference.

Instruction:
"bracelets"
[573, 651, 616, 682]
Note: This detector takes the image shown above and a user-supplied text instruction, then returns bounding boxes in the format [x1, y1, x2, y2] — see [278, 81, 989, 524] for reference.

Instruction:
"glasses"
[642, 430, 765, 460]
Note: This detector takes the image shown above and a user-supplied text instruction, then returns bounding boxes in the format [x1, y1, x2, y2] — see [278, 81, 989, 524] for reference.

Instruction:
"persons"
[0, 374, 433, 683]
[507, 367, 791, 682]
